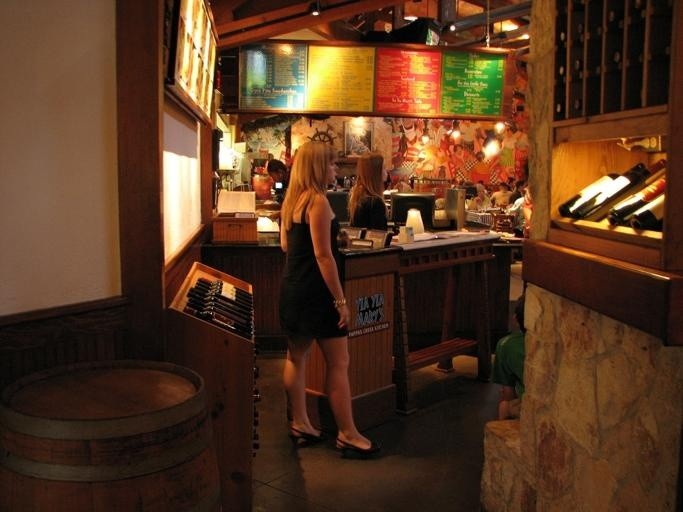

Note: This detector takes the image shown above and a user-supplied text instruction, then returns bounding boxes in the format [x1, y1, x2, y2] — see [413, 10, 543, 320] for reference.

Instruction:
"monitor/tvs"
[391, 193, 436, 232]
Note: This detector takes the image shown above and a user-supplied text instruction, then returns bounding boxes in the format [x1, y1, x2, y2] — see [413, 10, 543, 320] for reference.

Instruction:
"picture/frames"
[341, 120, 376, 157]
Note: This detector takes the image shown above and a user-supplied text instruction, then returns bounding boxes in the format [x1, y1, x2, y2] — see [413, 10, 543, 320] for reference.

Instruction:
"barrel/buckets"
[0, 360, 223, 512]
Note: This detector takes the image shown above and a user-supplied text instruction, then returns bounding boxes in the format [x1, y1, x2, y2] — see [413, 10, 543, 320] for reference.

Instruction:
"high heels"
[289, 425, 332, 446]
[335, 438, 384, 458]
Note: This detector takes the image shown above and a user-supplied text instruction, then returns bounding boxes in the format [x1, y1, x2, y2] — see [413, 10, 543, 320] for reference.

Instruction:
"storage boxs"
[213, 190, 260, 243]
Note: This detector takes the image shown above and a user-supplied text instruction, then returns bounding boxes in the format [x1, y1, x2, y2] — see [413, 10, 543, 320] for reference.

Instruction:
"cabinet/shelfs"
[546, 0, 682, 270]
[167, 261, 269, 510]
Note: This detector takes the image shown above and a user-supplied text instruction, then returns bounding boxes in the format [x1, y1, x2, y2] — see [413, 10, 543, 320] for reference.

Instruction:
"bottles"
[183, 278, 254, 341]
[557, 158, 666, 231]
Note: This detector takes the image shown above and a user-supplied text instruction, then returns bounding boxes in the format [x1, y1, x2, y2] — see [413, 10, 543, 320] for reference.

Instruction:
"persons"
[493, 294, 525, 420]
[267, 159, 290, 222]
[280, 141, 383, 458]
[465, 176, 532, 236]
[349, 152, 388, 231]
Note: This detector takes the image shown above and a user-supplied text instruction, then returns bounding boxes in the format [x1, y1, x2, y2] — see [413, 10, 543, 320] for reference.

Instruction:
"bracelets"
[334, 297, 346, 308]
